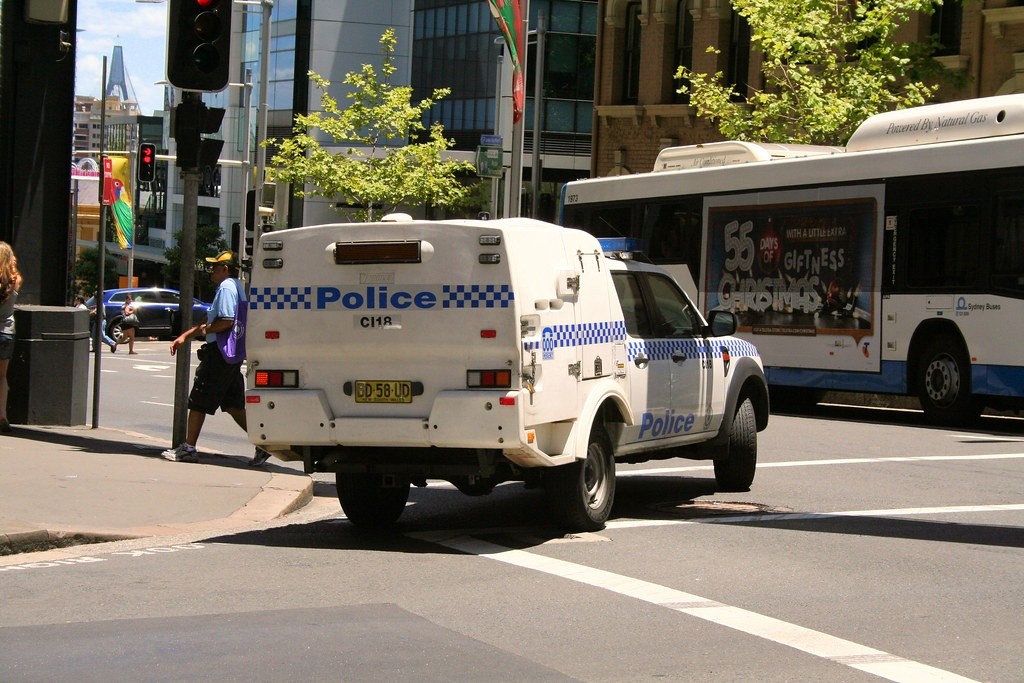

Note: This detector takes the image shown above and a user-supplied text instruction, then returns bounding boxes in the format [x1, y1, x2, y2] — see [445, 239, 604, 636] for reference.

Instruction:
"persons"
[75, 292, 139, 354]
[1, 241, 22, 433]
[161, 250, 271, 468]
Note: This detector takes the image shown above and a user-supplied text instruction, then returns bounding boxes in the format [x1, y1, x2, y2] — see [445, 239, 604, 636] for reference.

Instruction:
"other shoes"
[111, 344, 116, 353]
[129, 351, 137, 354]
[1, 417, 12, 432]
[89, 350, 95, 352]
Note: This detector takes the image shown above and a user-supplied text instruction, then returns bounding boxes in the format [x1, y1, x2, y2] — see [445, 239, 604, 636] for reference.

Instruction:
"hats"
[205, 251, 241, 271]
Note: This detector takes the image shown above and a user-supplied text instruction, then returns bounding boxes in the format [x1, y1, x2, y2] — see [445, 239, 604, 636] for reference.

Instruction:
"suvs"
[84, 286, 214, 343]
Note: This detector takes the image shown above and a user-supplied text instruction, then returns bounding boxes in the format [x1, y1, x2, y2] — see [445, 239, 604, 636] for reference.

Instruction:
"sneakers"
[160, 444, 199, 462]
[248, 450, 270, 467]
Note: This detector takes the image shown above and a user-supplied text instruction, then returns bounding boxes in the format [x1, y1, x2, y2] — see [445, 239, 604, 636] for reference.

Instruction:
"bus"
[557, 90, 1023, 427]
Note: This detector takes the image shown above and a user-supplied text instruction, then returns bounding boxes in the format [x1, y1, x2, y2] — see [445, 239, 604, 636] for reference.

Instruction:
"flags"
[104, 154, 132, 250]
[487, 0, 524, 123]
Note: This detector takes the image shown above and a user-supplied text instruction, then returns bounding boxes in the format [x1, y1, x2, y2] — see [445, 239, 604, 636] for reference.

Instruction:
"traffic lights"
[166, 0, 232, 93]
[138, 143, 156, 183]
[196, 105, 226, 135]
[195, 138, 224, 169]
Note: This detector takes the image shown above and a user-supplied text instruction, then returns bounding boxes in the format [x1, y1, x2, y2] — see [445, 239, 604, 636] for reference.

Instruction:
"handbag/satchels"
[216, 279, 247, 364]
[123, 313, 140, 326]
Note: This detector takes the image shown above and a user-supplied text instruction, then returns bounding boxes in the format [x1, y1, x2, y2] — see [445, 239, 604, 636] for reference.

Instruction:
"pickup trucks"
[244, 210, 770, 533]
